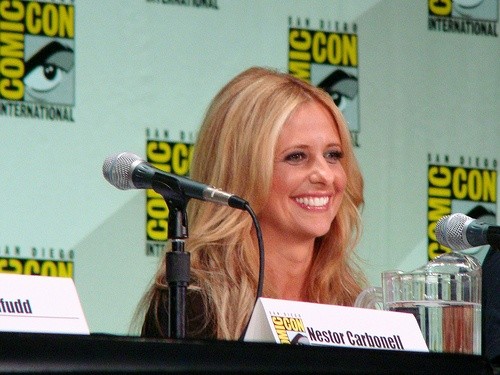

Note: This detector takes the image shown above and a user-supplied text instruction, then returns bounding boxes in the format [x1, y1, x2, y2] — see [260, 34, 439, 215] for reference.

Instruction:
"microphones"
[435, 214, 500, 251]
[102, 152, 250, 211]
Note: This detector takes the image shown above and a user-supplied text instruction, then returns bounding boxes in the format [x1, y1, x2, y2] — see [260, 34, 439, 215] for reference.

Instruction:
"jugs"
[353, 252, 485, 356]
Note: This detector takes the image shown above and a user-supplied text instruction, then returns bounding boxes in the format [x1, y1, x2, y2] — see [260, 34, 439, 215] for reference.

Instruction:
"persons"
[126, 66, 376, 342]
